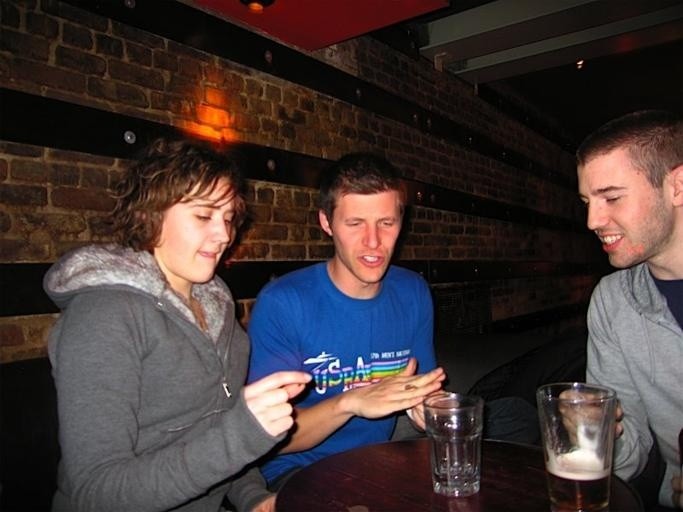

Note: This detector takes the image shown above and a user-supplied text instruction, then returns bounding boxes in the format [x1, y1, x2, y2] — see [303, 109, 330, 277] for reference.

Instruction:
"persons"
[557, 105, 682, 512]
[41, 138, 313, 512]
[244, 148, 448, 494]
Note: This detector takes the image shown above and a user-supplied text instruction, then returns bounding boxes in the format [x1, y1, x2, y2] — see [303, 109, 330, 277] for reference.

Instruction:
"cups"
[422, 392, 485, 498]
[535, 382, 618, 512]
